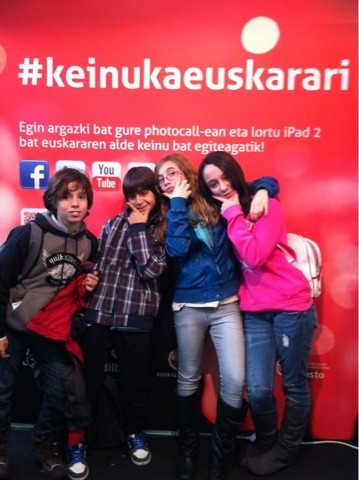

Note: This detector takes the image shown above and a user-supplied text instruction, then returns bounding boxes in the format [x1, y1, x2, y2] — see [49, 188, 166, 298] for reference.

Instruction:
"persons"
[59, 167, 167, 480]
[0, 168, 99, 480]
[154, 154, 280, 480]
[198, 151, 316, 475]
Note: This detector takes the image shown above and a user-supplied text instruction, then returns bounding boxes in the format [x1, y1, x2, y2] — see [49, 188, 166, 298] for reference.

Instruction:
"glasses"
[157, 169, 183, 184]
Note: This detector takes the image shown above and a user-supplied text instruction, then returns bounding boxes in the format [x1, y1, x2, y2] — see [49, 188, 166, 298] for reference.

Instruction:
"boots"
[174, 380, 203, 480]
[248, 407, 309, 476]
[240, 410, 279, 470]
[206, 396, 249, 480]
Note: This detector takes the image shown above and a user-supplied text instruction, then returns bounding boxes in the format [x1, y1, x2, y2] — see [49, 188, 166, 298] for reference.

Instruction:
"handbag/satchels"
[70, 308, 87, 343]
[284, 232, 323, 299]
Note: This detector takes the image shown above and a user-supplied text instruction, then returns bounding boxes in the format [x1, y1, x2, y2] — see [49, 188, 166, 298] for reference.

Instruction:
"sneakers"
[66, 442, 89, 480]
[30, 438, 68, 480]
[0, 445, 11, 478]
[128, 432, 152, 466]
